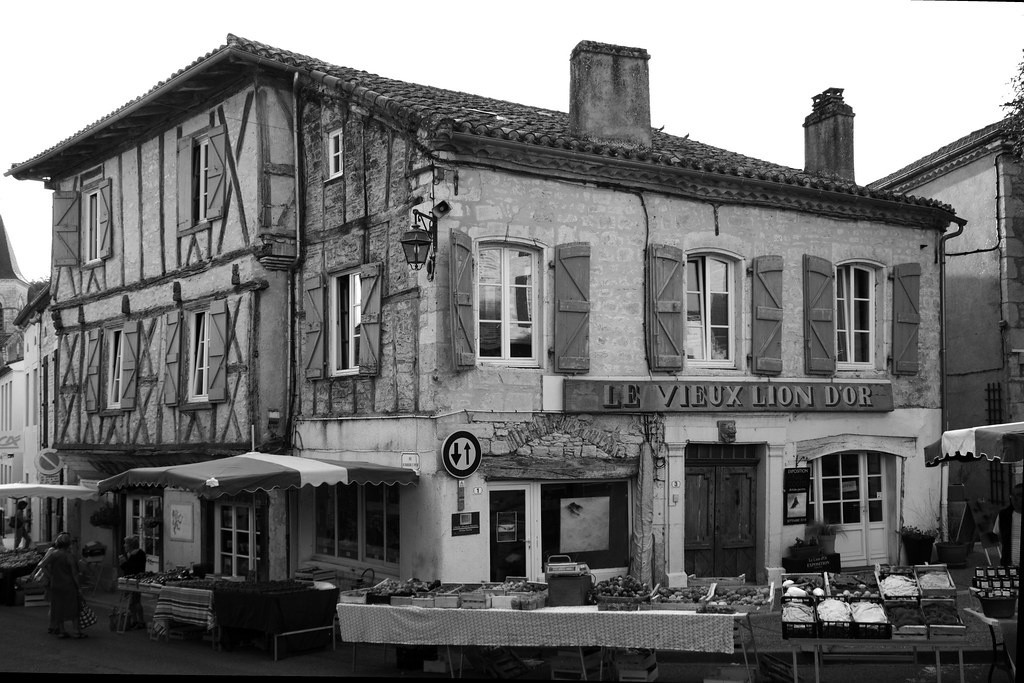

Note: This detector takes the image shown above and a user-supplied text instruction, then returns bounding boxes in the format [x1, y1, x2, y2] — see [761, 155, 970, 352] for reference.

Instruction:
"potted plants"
[807, 516, 849, 557]
[932, 508, 974, 565]
[788, 537, 820, 559]
[893, 522, 938, 565]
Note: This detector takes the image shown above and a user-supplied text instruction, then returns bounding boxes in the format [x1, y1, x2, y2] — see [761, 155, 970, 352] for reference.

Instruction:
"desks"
[963, 608, 1019, 683]
[338, 602, 762, 683]
[83, 560, 107, 596]
[110, 575, 340, 659]
[785, 636, 966, 683]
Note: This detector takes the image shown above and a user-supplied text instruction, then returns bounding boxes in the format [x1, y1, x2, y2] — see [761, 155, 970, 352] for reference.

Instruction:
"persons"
[39, 532, 71, 634]
[37, 534, 87, 638]
[118, 536, 147, 630]
[14, 501, 32, 549]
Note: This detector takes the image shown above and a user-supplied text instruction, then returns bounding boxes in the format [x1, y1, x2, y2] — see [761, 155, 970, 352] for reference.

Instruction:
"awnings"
[922, 418, 1024, 468]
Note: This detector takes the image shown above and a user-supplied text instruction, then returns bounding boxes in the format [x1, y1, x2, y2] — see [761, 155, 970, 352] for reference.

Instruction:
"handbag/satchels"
[33, 567, 51, 587]
[79, 601, 98, 630]
[109, 606, 126, 630]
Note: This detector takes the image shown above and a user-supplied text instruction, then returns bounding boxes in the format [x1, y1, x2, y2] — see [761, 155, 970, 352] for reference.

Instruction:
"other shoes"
[56, 632, 70, 638]
[130, 622, 137, 628]
[134, 623, 146, 629]
[73, 632, 88, 639]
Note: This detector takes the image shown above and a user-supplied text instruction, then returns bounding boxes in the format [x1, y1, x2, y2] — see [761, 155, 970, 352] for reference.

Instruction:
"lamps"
[397, 208, 438, 272]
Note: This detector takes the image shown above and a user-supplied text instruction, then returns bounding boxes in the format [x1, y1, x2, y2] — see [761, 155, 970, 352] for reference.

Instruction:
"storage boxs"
[337, 575, 552, 610]
[780, 559, 970, 641]
[616, 648, 660, 683]
[596, 575, 775, 614]
[423, 660, 462, 673]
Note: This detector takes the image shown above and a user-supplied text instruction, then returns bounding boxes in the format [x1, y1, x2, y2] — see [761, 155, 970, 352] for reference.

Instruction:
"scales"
[294, 566, 337, 581]
[544, 561, 591, 577]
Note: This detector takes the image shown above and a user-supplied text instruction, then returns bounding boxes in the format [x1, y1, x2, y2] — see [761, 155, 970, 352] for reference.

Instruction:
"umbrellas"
[96, 425, 418, 582]
[0, 472, 99, 500]
